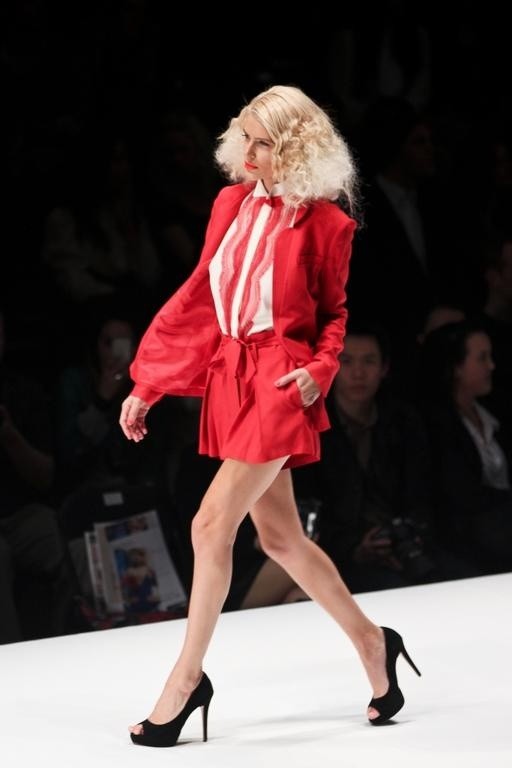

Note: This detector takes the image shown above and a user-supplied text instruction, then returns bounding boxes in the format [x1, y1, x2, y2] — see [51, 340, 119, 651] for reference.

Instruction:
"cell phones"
[110, 338, 133, 390]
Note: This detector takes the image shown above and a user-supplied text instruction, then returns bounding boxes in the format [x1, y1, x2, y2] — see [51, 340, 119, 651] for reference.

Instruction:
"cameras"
[373, 514, 419, 553]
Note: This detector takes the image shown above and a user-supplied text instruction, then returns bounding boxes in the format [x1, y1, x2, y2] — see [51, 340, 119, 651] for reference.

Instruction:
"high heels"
[131, 671, 214, 748]
[367, 626, 422, 726]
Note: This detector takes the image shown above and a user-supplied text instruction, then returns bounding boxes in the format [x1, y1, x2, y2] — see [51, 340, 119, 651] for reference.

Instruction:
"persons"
[114, 82, 423, 751]
[1, 101, 511, 645]
